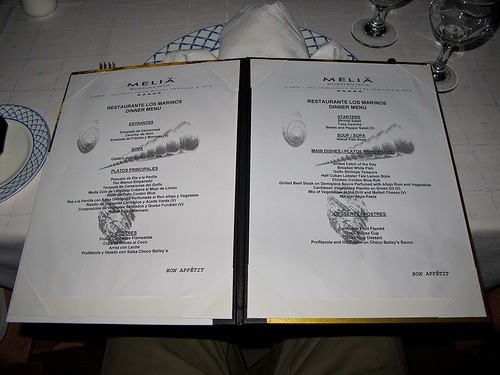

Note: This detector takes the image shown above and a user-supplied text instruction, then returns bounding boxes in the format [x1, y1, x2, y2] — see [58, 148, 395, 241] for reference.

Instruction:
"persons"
[1, 288, 405, 375]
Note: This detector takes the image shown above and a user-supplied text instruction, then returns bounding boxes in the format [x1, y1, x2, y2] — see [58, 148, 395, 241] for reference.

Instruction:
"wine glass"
[349, 0, 413, 49]
[423, 0, 495, 94]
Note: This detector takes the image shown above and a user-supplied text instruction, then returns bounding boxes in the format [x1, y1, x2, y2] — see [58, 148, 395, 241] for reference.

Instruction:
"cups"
[22, 0, 58, 18]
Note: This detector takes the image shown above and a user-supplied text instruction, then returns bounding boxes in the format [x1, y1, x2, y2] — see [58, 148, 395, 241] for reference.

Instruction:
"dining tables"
[2, 0, 499, 298]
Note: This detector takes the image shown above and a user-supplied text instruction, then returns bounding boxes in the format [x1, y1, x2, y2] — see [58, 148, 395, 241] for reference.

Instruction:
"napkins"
[163, 2, 352, 61]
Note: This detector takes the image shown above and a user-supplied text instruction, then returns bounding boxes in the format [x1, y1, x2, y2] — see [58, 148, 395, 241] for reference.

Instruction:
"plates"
[0, 101, 51, 202]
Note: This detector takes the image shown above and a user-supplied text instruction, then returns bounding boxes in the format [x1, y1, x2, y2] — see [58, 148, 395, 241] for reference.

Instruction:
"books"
[6, 57, 493, 339]
